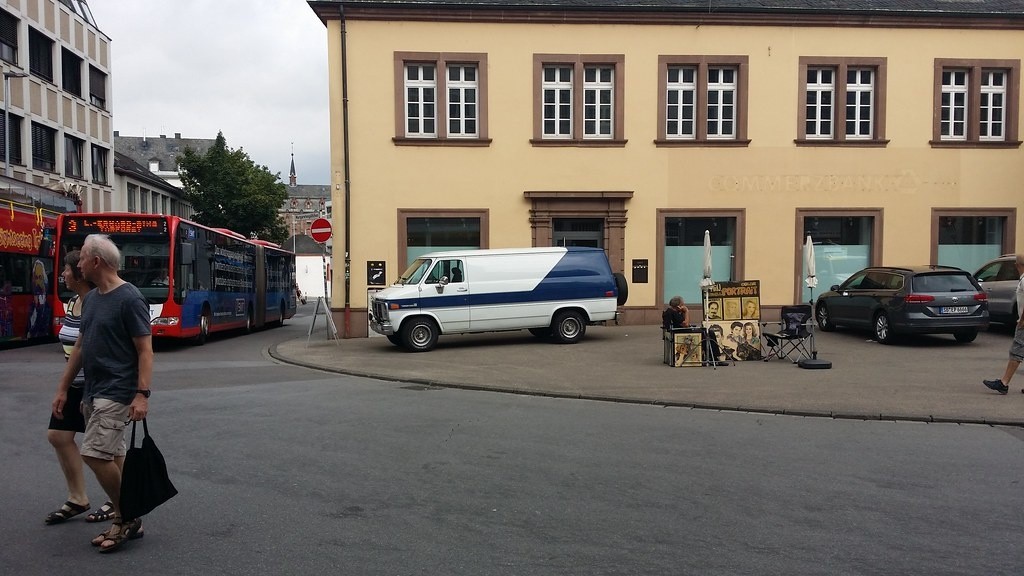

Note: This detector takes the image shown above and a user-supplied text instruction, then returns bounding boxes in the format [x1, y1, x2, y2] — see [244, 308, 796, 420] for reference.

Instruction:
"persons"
[982, 253, 1024, 394]
[52, 234, 155, 554]
[43, 249, 115, 524]
[664, 295, 729, 366]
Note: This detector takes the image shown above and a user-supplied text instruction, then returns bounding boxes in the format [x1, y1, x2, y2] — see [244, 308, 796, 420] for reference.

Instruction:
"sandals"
[98, 518, 144, 554]
[90, 530, 111, 546]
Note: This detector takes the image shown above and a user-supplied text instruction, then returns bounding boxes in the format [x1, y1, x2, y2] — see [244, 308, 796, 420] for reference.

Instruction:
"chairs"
[660, 307, 718, 367]
[451, 268, 462, 283]
[762, 305, 817, 365]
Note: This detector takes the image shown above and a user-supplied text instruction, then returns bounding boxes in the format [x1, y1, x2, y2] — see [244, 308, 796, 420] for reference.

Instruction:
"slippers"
[45, 501, 90, 525]
[85, 501, 116, 523]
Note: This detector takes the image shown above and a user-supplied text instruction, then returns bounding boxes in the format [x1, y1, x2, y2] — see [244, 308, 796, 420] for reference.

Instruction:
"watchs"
[135, 389, 151, 398]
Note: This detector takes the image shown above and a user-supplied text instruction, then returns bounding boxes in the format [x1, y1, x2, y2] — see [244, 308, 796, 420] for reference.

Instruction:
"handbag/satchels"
[119, 417, 178, 525]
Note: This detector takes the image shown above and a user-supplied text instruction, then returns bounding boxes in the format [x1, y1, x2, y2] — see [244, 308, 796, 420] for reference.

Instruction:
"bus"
[0, 173, 83, 343]
[37, 211, 297, 346]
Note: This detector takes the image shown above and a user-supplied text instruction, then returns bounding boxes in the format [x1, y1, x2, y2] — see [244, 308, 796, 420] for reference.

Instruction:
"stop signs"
[310, 219, 332, 244]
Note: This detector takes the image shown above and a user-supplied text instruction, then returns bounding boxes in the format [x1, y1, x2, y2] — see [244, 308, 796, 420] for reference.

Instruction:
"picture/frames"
[674, 333, 702, 367]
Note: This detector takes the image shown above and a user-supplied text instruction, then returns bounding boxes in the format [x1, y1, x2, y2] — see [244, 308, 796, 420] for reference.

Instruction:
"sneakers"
[983, 379, 1009, 395]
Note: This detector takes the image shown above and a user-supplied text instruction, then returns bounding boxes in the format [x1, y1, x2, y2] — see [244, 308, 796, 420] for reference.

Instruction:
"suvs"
[972, 253, 1020, 330]
[368, 246, 629, 353]
[814, 263, 989, 346]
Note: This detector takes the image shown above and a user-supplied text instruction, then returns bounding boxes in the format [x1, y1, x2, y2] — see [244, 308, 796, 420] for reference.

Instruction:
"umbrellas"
[805, 231, 818, 349]
[698, 230, 715, 364]
